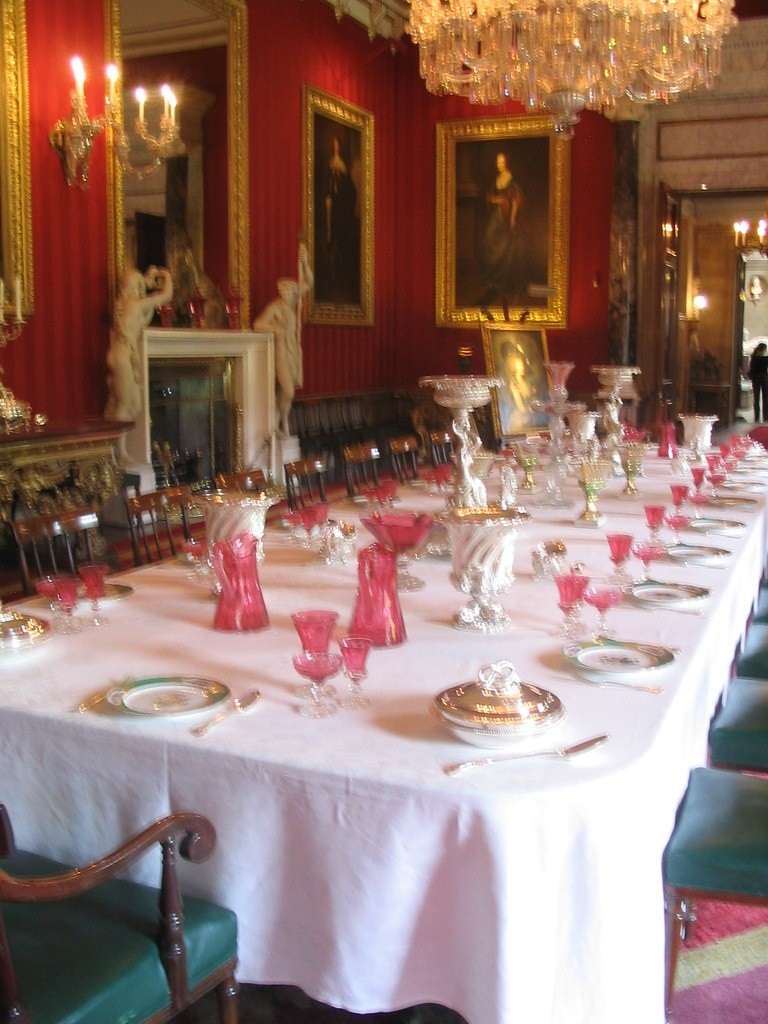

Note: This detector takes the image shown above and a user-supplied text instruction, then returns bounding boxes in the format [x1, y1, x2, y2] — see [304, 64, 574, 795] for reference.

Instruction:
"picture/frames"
[300, 81, 376, 330]
[436, 115, 571, 332]
[480, 321, 559, 443]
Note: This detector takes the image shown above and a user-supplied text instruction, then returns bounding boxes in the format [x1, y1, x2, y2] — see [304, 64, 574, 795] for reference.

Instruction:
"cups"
[531, 539, 567, 580]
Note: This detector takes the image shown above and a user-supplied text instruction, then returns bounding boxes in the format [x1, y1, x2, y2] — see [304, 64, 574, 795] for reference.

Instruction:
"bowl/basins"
[428, 661, 568, 751]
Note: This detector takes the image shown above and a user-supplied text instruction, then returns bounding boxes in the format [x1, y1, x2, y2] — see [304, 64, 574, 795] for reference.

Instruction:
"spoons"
[443, 732, 609, 774]
[192, 690, 261, 737]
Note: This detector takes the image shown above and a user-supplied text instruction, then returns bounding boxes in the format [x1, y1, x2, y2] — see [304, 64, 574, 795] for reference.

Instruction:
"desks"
[0, 438, 768, 1024]
[0, 417, 138, 572]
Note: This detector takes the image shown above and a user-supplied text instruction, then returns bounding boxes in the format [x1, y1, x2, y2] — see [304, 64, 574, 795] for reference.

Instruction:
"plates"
[746, 456, 768, 461]
[709, 495, 759, 508]
[664, 518, 748, 564]
[562, 585, 708, 673]
[721, 479, 766, 492]
[734, 466, 768, 473]
[110, 676, 230, 716]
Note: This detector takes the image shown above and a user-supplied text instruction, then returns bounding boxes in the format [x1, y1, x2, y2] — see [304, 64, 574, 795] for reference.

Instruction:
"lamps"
[402, 0, 739, 128]
[694, 288, 707, 311]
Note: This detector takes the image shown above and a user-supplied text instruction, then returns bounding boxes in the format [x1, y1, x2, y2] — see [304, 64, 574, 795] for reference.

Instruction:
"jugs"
[659, 422, 677, 458]
[212, 533, 269, 633]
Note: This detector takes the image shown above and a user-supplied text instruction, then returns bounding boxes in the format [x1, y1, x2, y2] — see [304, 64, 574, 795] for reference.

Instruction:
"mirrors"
[107, 0, 250, 333]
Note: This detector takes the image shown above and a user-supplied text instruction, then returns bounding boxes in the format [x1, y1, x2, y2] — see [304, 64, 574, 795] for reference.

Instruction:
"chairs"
[666, 585, 768, 1008]
[0, 799, 240, 1024]
[7, 430, 460, 594]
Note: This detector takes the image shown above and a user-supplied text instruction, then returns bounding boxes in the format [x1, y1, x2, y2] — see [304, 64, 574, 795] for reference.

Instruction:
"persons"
[104, 269, 173, 468]
[750, 343, 768, 423]
[186, 253, 229, 328]
[255, 249, 314, 435]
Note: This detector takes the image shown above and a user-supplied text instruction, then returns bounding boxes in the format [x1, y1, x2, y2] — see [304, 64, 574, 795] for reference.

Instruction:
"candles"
[69, 54, 179, 128]
[733, 219, 767, 248]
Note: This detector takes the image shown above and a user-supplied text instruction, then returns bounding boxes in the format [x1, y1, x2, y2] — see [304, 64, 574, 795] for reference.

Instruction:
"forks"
[76, 676, 133, 713]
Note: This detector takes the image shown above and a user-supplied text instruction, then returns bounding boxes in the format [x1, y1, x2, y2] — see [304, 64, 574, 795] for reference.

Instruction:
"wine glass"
[283, 465, 532, 632]
[645, 501, 688, 543]
[519, 454, 641, 527]
[605, 532, 663, 585]
[293, 636, 372, 717]
[182, 544, 202, 579]
[34, 576, 84, 634]
[555, 574, 622, 643]
[692, 433, 753, 497]
[79, 565, 110, 626]
[670, 481, 710, 519]
[292, 609, 339, 700]
